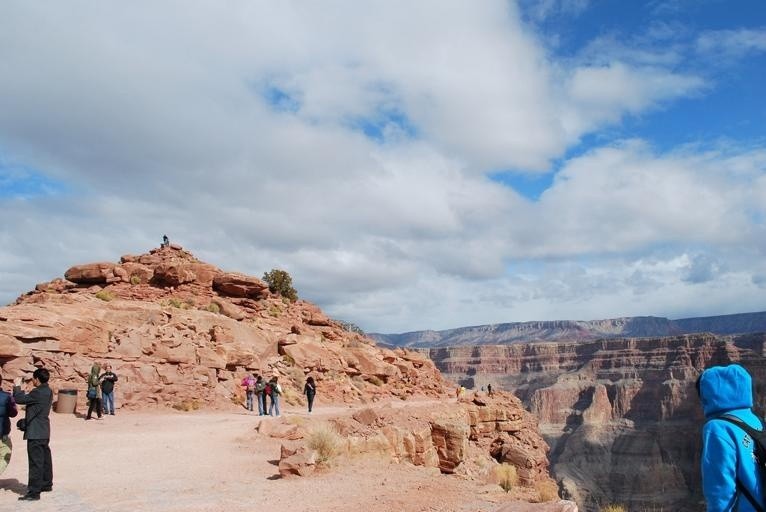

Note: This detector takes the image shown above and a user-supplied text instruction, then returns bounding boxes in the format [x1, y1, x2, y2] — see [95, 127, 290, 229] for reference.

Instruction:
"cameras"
[21, 378, 25, 382]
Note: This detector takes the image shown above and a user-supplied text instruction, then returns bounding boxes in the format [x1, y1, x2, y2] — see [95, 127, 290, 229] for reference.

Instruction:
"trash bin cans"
[56, 389, 78, 413]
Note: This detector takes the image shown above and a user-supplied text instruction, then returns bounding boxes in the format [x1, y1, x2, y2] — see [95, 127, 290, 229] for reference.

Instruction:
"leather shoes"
[18, 491, 40, 501]
[40, 486, 52, 492]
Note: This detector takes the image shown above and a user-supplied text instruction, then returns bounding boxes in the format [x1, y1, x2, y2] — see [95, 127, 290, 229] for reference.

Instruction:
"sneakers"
[258, 412, 281, 418]
[85, 415, 107, 421]
[105, 412, 115, 416]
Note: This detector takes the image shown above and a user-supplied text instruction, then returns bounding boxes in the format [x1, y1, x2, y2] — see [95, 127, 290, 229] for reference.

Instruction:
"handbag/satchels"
[86, 390, 97, 401]
[16, 418, 27, 432]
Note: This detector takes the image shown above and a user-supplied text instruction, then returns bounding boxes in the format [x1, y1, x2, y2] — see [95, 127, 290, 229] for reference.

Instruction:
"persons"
[3, 367, 53, 501]
[97, 365, 118, 415]
[254, 375, 268, 416]
[162, 234, 168, 244]
[83, 365, 105, 420]
[267, 376, 283, 417]
[459, 384, 465, 397]
[455, 388, 460, 396]
[0, 375, 18, 481]
[303, 376, 317, 411]
[694, 362, 765, 511]
[488, 383, 492, 394]
[243, 373, 257, 411]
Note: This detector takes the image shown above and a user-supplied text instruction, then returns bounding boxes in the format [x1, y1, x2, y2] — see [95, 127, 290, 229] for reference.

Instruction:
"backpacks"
[246, 384, 254, 392]
[265, 382, 277, 394]
[255, 384, 264, 395]
